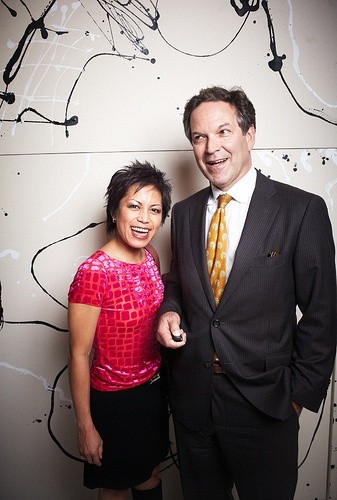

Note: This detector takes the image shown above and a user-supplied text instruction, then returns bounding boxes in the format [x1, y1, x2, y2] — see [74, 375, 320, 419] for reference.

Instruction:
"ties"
[206, 194, 233, 309]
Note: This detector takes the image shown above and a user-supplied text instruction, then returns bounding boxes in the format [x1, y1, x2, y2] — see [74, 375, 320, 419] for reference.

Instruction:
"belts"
[213, 353, 225, 374]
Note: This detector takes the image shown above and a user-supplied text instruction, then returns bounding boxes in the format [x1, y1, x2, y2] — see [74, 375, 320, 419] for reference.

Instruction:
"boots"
[131, 479, 163, 500]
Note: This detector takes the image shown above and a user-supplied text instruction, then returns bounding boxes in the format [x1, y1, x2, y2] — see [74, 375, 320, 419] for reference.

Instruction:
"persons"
[153, 86, 337, 500]
[65, 160, 174, 499]
